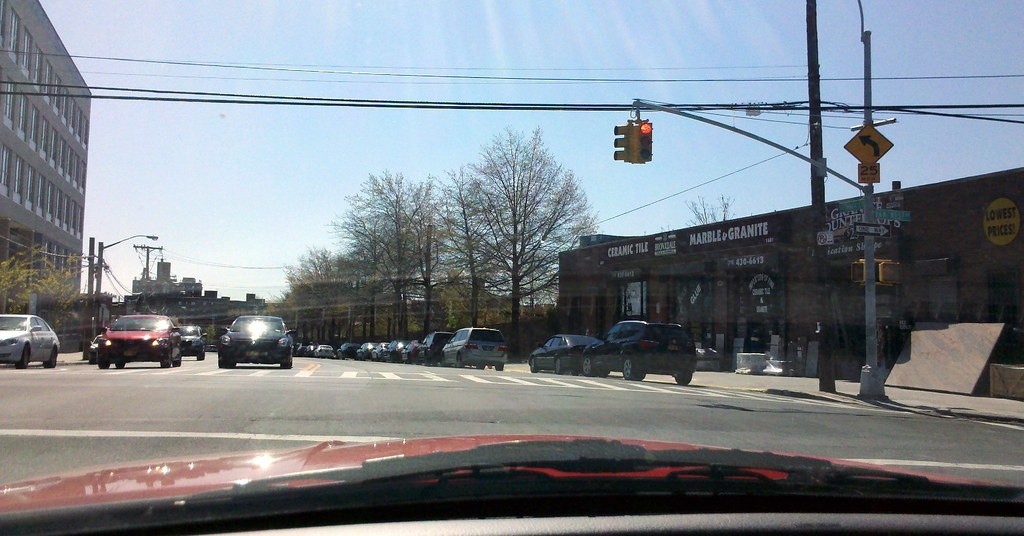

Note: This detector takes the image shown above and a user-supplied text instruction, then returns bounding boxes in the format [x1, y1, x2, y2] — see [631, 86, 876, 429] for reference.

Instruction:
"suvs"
[442, 327, 509, 371]
[581, 320, 696, 384]
[417, 331, 456, 367]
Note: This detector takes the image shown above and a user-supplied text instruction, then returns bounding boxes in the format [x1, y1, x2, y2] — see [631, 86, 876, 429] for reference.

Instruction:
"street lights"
[96, 234, 159, 307]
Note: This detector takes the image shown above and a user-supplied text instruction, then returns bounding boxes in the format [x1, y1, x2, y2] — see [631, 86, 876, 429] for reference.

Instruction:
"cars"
[217, 314, 298, 369]
[177, 324, 205, 361]
[383, 340, 412, 363]
[401, 340, 427, 365]
[90, 334, 103, 365]
[292, 341, 389, 362]
[528, 333, 602, 375]
[98, 314, 180, 369]
[0, 314, 60, 369]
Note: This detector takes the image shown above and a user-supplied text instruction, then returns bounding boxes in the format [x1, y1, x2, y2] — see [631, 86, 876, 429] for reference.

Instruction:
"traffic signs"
[837, 201, 872, 211]
[853, 222, 891, 237]
[864, 209, 911, 221]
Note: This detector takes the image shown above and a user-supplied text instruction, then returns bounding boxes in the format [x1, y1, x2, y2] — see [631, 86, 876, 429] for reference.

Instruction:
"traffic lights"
[850, 259, 866, 285]
[879, 260, 905, 285]
[614, 118, 633, 163]
[633, 119, 654, 164]
